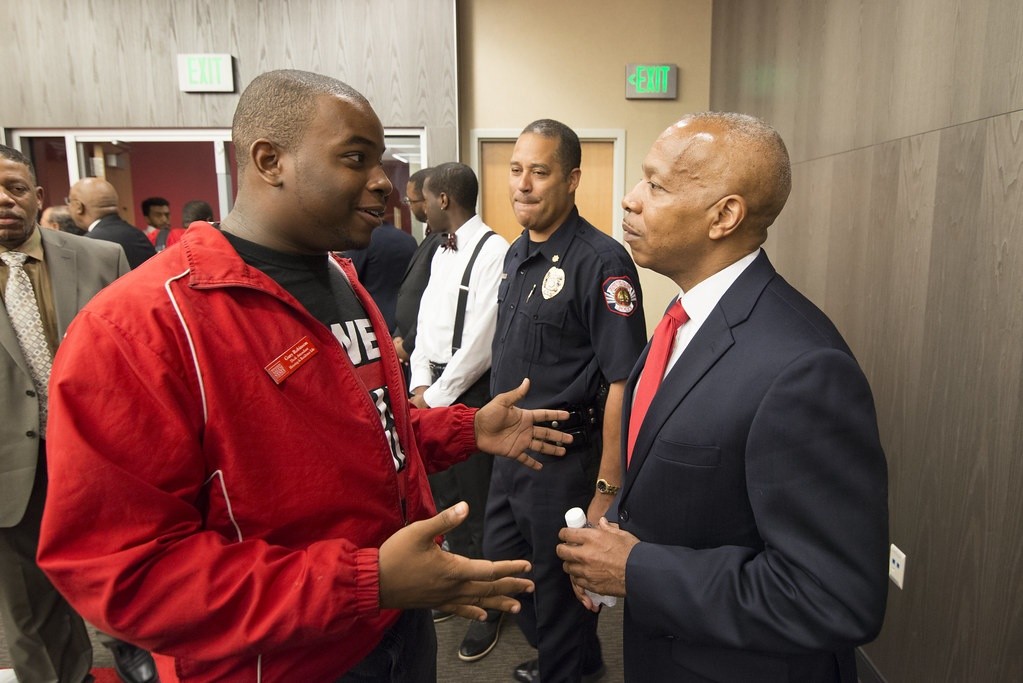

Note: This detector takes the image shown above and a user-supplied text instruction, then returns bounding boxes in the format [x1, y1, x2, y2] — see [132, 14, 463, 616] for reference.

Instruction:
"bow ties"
[438, 231, 459, 252]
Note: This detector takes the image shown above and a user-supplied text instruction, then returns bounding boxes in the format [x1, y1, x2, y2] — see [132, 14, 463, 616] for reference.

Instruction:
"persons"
[333, 120, 647, 683]
[0, 144, 158, 683]
[40, 177, 214, 269]
[555, 112, 890, 683]
[36, 67, 572, 683]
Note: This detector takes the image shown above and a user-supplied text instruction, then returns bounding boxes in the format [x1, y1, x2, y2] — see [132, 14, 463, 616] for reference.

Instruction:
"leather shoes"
[112, 640, 159, 683]
[459, 607, 504, 662]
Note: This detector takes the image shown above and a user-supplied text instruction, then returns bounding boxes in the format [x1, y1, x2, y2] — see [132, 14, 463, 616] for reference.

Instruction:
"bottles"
[564, 507, 617, 607]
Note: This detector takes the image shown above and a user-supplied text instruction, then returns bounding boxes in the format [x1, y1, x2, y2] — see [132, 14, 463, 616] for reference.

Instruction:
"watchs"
[596, 478, 620, 495]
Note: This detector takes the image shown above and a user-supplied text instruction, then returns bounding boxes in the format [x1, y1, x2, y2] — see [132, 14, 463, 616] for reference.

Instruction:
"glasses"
[401, 196, 423, 207]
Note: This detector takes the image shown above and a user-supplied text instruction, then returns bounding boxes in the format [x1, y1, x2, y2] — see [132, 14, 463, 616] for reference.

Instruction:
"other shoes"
[513, 658, 606, 682]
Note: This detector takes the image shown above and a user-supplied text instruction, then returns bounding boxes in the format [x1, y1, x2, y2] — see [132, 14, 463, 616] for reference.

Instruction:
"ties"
[0, 250, 54, 437]
[625, 298, 692, 476]
